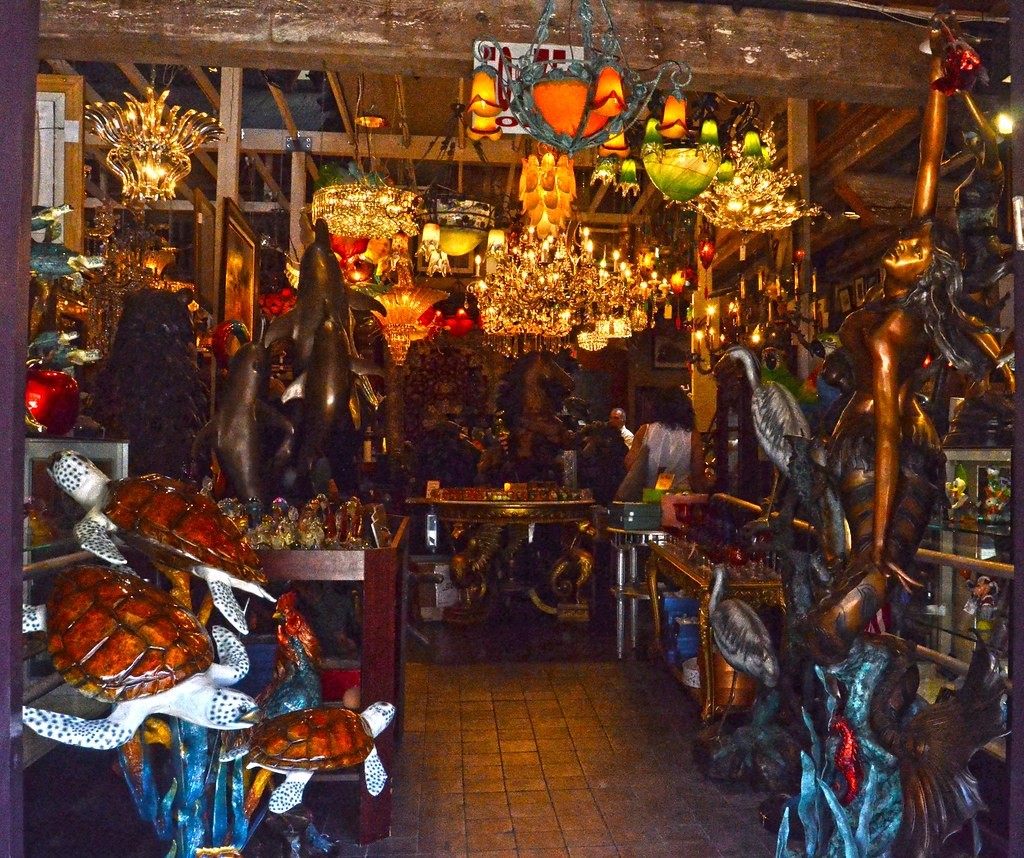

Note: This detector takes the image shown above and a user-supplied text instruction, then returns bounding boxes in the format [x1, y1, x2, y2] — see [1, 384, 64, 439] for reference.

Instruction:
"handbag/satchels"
[615, 423, 651, 503]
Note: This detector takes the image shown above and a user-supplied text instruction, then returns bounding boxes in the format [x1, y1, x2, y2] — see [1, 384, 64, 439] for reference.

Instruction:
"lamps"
[591, 90, 766, 206]
[686, 118, 831, 235]
[414, 222, 441, 262]
[474, 0, 695, 161]
[359, 236, 450, 366]
[696, 246, 831, 367]
[409, 306, 446, 341]
[467, 231, 692, 357]
[416, 186, 516, 258]
[515, 142, 579, 241]
[87, 85, 227, 204]
[307, 163, 425, 240]
[429, 250, 453, 277]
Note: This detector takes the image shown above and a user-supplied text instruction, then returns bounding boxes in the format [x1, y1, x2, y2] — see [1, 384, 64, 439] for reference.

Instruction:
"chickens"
[272, 590, 323, 679]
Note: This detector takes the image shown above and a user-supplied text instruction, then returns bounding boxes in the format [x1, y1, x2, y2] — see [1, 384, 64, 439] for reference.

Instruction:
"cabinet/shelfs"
[21, 431, 128, 767]
[606, 524, 669, 661]
[882, 437, 1012, 836]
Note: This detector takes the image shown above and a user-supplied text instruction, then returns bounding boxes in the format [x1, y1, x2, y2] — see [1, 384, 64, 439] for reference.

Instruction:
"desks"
[405, 491, 596, 624]
[647, 533, 784, 754]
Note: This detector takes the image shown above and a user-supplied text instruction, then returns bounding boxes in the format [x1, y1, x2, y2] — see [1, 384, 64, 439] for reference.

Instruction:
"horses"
[498, 349, 576, 453]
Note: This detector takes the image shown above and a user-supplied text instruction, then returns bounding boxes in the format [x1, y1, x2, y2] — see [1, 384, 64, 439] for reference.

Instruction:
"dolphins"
[194, 217, 389, 506]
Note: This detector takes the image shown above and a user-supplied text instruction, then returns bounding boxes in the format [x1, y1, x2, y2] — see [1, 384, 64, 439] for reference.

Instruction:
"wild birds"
[712, 346, 812, 528]
[706, 563, 778, 745]
[263, 637, 322, 719]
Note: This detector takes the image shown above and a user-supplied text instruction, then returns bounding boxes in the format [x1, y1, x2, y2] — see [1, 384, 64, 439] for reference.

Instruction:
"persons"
[625, 385, 710, 501]
[610, 408, 634, 450]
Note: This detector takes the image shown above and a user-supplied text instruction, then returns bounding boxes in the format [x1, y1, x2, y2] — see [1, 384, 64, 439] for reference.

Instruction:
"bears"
[83, 286, 212, 485]
